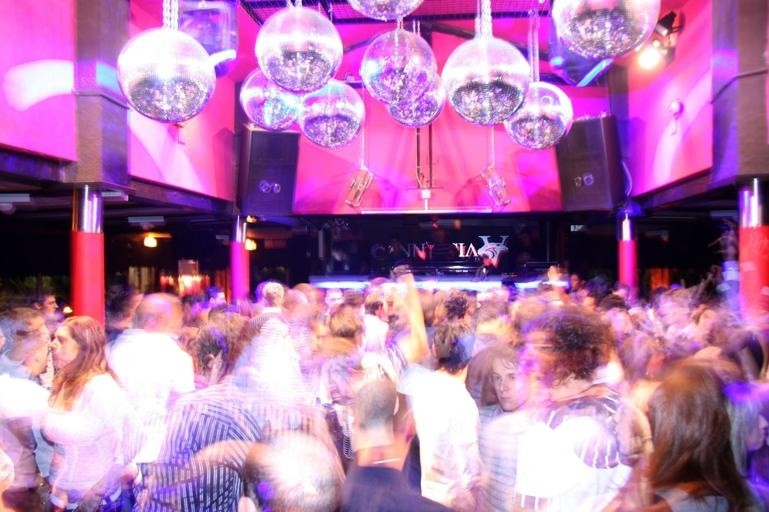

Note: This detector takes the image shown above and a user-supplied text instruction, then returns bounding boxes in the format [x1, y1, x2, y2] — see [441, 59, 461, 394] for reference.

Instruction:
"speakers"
[552, 111, 626, 213]
[236, 122, 301, 217]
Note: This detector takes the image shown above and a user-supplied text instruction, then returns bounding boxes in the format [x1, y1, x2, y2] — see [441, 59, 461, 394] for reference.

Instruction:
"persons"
[0, 256, 768, 510]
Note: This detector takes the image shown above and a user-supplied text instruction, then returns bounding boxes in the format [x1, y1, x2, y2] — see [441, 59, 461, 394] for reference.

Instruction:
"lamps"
[654, 9, 682, 38]
